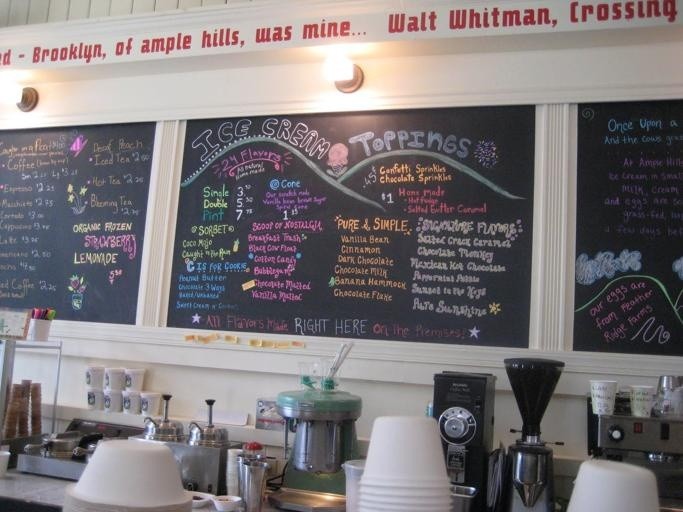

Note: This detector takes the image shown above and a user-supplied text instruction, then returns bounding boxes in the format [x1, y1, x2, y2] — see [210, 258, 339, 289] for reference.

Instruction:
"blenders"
[430, 371, 498, 510]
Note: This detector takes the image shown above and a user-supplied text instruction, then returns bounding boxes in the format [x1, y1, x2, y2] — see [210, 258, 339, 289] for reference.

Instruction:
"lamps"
[321, 52, 363, 93]
[6, 83, 38, 112]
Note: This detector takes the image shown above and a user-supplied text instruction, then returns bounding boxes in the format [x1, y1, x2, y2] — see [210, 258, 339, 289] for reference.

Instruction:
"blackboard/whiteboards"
[1, 120, 157, 325]
[165, 103, 536, 348]
[573, 99, 682, 358]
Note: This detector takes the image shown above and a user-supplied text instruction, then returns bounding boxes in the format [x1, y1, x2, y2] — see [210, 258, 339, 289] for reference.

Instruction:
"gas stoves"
[17, 421, 150, 484]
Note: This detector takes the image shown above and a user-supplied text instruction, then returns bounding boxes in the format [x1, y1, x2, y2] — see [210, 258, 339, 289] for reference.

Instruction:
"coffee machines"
[500, 359, 563, 512]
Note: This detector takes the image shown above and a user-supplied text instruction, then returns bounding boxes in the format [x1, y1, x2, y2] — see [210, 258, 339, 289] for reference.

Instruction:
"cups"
[569, 454, 663, 512]
[61, 441, 192, 512]
[226, 449, 268, 512]
[590, 380, 654, 420]
[81, 363, 161, 418]
[354, 419, 452, 512]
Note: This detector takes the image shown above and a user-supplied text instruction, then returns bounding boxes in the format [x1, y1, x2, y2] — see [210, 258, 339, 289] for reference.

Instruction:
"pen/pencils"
[33, 307, 56, 320]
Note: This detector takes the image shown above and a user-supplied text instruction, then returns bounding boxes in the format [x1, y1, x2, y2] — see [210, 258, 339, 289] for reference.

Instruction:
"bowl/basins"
[182, 492, 241, 510]
